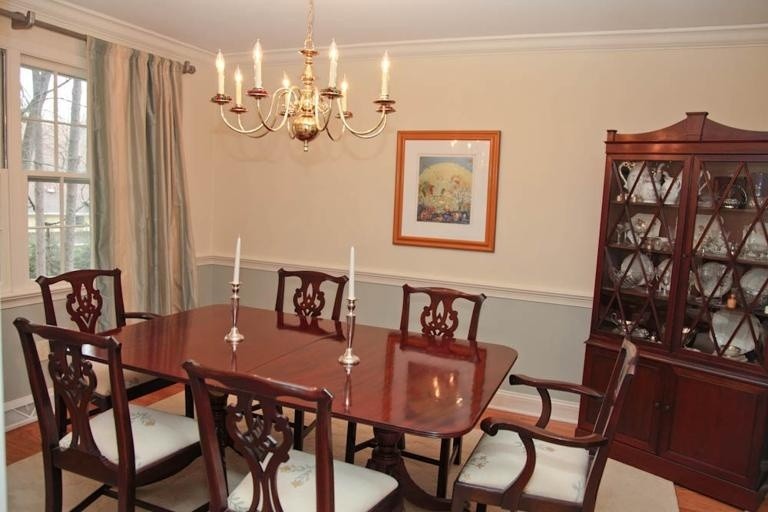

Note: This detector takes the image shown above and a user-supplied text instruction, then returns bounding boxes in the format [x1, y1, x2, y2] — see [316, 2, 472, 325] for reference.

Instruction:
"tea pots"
[618, 160, 663, 198]
[659, 172, 682, 204]
[639, 173, 661, 204]
[698, 169, 710, 195]
[717, 181, 747, 209]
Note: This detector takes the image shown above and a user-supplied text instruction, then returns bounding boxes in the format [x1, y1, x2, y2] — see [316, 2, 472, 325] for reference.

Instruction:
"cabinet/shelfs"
[575, 109, 768, 512]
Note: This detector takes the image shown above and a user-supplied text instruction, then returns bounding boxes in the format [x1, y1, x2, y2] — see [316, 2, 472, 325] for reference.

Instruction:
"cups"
[614, 224, 624, 249]
[619, 223, 633, 250]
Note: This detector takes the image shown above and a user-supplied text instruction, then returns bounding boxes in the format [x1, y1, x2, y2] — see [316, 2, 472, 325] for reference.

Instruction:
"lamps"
[211, 0, 397, 154]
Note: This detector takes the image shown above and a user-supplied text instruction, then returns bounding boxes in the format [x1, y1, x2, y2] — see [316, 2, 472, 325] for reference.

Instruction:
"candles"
[231, 233, 242, 283]
[347, 244, 358, 301]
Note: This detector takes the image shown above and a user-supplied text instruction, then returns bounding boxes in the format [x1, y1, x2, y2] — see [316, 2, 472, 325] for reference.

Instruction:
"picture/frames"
[395, 130, 502, 252]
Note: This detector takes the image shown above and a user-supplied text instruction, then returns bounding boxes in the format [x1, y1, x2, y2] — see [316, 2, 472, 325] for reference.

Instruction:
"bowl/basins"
[720, 345, 741, 357]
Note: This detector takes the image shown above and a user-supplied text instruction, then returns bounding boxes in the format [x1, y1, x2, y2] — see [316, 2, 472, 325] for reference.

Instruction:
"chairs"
[344, 284, 488, 498]
[11, 316, 221, 512]
[36, 267, 194, 446]
[451, 336, 638, 511]
[182, 359, 404, 511]
[227, 267, 349, 451]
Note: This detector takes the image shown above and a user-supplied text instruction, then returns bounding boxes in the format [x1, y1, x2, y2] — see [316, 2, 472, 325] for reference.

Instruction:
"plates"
[656, 257, 694, 295]
[674, 213, 724, 253]
[630, 212, 662, 241]
[740, 268, 767, 309]
[694, 262, 734, 298]
[741, 221, 767, 261]
[619, 252, 654, 287]
[709, 307, 763, 357]
